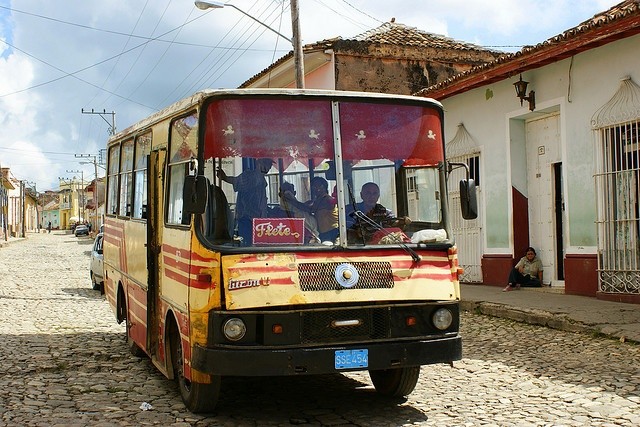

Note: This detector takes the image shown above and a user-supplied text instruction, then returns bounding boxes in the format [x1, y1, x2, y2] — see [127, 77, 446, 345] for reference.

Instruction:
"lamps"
[513, 74, 535, 111]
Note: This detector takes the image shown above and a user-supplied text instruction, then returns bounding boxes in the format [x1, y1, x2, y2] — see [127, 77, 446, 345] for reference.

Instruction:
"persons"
[284, 176, 339, 243]
[345, 181, 413, 243]
[217, 158, 276, 246]
[278, 181, 319, 237]
[502, 247, 545, 292]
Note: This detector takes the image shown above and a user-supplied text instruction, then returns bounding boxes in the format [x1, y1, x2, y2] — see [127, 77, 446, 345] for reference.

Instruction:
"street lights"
[194, 0, 295, 47]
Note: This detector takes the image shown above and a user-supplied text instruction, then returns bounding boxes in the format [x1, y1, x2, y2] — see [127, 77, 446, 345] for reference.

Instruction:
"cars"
[91, 233, 106, 291]
[74, 225, 89, 237]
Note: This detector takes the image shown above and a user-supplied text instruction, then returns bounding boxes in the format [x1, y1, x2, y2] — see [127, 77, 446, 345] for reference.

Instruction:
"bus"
[103, 89, 477, 413]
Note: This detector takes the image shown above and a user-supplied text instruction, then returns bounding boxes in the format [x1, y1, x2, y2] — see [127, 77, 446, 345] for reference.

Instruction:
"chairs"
[201, 184, 239, 244]
